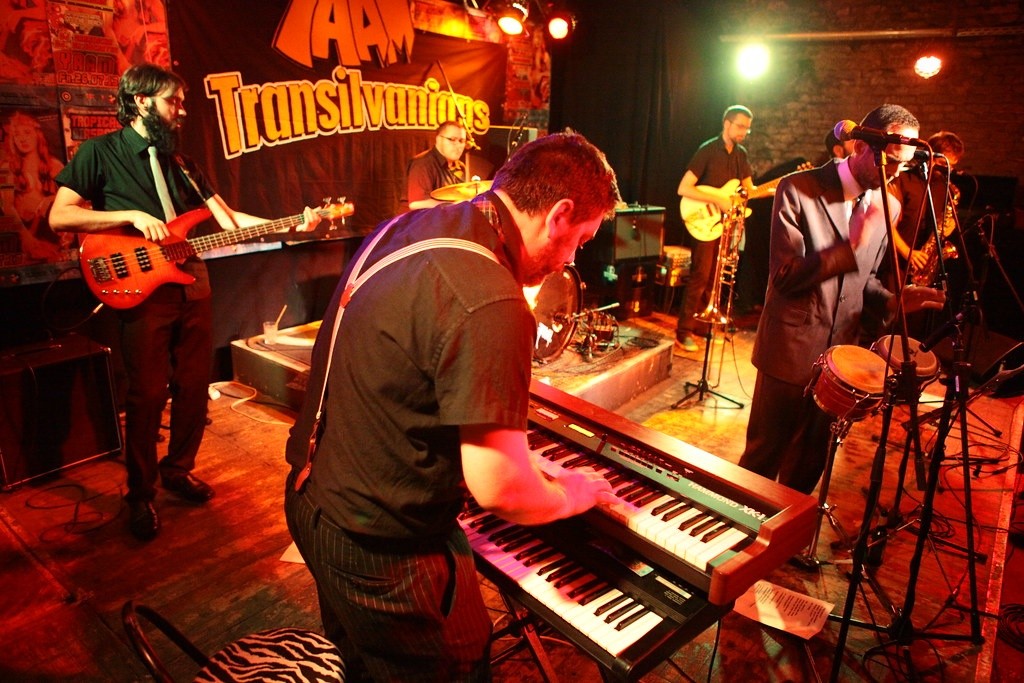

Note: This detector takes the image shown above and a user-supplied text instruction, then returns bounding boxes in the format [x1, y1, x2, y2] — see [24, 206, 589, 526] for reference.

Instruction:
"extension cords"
[207, 387, 221, 400]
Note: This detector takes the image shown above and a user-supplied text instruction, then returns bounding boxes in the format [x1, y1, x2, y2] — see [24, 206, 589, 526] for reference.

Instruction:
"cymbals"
[430, 180, 498, 203]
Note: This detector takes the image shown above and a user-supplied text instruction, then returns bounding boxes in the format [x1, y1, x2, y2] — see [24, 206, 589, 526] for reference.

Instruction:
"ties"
[848, 193, 865, 246]
[147, 145, 187, 266]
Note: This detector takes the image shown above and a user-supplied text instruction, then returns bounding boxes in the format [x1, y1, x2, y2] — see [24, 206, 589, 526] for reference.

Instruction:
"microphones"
[632, 220, 637, 238]
[914, 149, 944, 159]
[933, 164, 972, 176]
[964, 214, 991, 234]
[555, 312, 573, 322]
[512, 116, 528, 147]
[834, 119, 929, 147]
[919, 308, 974, 353]
[464, 141, 481, 151]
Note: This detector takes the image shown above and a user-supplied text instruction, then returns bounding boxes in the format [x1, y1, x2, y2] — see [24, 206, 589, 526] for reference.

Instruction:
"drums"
[522, 263, 585, 366]
[872, 334, 940, 396]
[809, 343, 894, 425]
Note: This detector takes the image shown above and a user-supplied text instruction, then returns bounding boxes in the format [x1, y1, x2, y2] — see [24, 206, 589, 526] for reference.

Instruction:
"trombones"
[691, 185, 751, 391]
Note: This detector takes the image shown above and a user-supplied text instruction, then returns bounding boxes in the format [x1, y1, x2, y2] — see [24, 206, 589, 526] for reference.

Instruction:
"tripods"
[671, 322, 744, 409]
[567, 302, 620, 362]
[826, 146, 1024, 683]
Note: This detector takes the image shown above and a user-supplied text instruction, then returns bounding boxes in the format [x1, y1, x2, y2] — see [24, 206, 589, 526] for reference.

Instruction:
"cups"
[263, 321, 279, 345]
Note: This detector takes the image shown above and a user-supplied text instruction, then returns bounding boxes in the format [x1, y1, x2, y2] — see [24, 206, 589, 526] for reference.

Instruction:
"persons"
[48, 64, 323, 541]
[0, 0, 145, 260]
[406, 123, 472, 206]
[735, 103, 966, 571]
[676, 105, 778, 353]
[286, 131, 624, 683]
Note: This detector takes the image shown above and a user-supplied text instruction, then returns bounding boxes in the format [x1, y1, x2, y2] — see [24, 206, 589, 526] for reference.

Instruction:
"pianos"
[449, 377, 825, 682]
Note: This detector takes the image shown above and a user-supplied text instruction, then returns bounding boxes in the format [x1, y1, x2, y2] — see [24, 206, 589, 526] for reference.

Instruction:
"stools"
[656, 245, 694, 313]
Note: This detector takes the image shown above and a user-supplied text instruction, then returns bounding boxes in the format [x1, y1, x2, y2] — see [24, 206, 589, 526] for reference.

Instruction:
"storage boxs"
[0, 333, 122, 490]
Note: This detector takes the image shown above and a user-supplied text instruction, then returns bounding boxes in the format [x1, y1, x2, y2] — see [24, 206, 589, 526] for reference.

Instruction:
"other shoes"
[691, 333, 724, 344]
[675, 336, 699, 351]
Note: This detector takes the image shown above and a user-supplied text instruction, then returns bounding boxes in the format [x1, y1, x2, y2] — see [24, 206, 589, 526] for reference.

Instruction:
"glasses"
[440, 134, 467, 144]
[732, 121, 752, 134]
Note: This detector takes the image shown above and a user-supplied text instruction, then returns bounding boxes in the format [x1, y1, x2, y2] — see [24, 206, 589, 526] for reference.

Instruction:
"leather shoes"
[128, 494, 160, 540]
[158, 456, 215, 506]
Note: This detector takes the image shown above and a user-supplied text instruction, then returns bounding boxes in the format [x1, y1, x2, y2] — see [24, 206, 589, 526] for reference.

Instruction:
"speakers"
[0, 332, 121, 492]
[586, 205, 665, 265]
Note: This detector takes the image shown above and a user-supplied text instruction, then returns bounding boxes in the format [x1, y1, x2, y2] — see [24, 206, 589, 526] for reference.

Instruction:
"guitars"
[680, 159, 815, 242]
[79, 193, 356, 311]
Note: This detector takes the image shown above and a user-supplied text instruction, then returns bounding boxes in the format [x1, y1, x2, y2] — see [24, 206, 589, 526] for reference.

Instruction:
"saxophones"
[911, 172, 960, 287]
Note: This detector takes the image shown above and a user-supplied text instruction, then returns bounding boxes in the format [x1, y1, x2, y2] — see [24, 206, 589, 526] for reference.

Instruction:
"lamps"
[488, 0, 582, 52]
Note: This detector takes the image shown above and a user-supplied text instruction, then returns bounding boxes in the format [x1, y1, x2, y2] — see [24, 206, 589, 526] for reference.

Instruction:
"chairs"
[120, 599, 348, 683]
